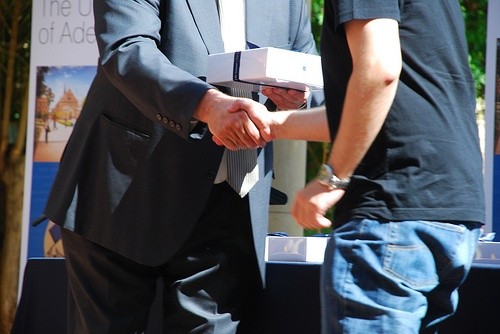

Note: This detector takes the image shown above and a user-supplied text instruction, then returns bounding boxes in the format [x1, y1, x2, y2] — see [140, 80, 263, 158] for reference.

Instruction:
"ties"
[217, 0, 260, 199]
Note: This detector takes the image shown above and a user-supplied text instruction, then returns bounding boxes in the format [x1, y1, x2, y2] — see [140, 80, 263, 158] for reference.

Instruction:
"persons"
[43, 0, 326, 334]
[212, 0, 487, 334]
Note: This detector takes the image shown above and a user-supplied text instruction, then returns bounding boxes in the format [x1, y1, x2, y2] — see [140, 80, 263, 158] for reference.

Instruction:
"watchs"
[317, 162, 351, 191]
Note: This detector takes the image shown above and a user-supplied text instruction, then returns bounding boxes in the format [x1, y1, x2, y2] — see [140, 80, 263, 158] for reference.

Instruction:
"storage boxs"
[205, 46, 323, 93]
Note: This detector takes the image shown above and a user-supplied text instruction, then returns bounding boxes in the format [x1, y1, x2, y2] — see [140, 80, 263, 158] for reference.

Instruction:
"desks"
[6, 256, 500, 334]
